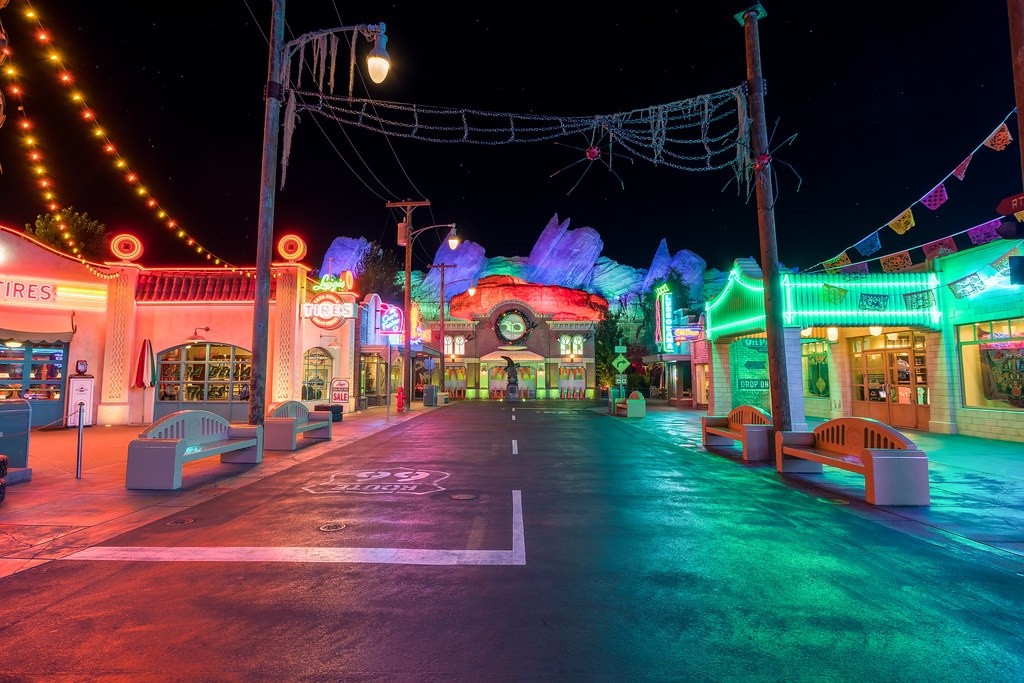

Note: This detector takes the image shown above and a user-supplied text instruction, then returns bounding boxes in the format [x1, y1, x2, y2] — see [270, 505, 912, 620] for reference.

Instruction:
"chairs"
[650, 386, 656, 398]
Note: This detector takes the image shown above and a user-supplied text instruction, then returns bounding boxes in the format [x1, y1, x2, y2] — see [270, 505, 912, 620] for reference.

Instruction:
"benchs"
[615, 391, 646, 417]
[775, 417, 930, 506]
[263, 400, 332, 451]
[125, 410, 263, 489]
[438, 392, 449, 404]
[702, 405, 774, 461]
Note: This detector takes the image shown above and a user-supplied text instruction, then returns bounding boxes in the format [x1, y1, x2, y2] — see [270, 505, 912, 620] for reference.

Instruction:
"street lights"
[250, 18, 393, 427]
[403, 224, 461, 411]
[440, 278, 476, 394]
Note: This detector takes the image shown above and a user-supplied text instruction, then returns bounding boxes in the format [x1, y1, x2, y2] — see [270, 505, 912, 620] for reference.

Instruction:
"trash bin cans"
[608, 386, 625, 414]
[0, 399, 32, 468]
[422, 384, 437, 406]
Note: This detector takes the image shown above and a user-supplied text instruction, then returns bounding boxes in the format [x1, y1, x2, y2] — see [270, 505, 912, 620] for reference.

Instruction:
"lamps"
[185, 326, 210, 342]
[320, 334, 344, 349]
[828, 328, 838, 341]
[570, 354, 574, 361]
[801, 327, 812, 336]
[887, 333, 898, 341]
[481, 364, 487, 375]
[869, 326, 882, 336]
[539, 364, 544, 376]
[451, 353, 456, 362]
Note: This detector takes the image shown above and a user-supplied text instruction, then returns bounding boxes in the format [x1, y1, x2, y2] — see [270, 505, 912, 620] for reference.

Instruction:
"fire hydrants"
[394, 387, 408, 413]
